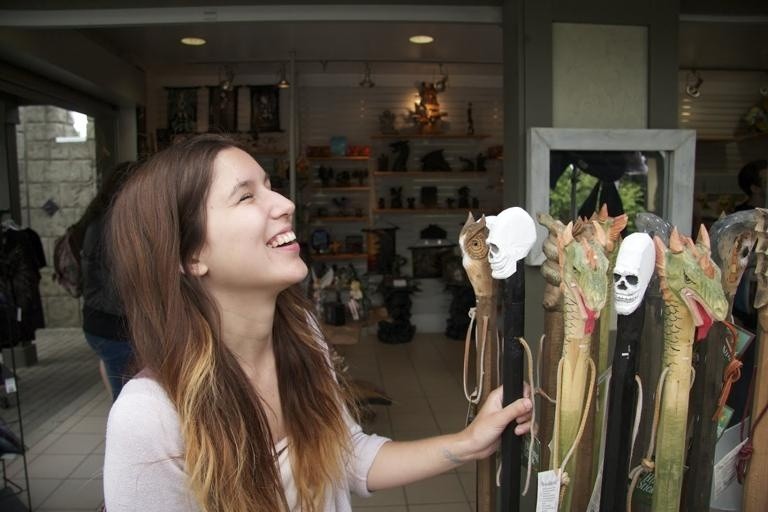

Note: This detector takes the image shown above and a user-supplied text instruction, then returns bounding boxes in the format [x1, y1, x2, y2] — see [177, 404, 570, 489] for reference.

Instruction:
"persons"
[732, 160, 768, 214]
[102, 133, 538, 512]
[74, 153, 134, 400]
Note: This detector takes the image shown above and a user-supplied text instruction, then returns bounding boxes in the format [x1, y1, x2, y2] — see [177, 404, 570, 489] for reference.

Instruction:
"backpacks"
[53, 224, 105, 297]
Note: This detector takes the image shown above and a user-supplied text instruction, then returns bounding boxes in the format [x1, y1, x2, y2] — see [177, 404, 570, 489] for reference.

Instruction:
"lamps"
[211, 58, 449, 95]
[682, 65, 707, 99]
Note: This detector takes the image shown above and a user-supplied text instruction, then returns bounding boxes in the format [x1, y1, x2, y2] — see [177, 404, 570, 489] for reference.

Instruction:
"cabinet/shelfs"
[305, 155, 372, 263]
[372, 127, 491, 219]
